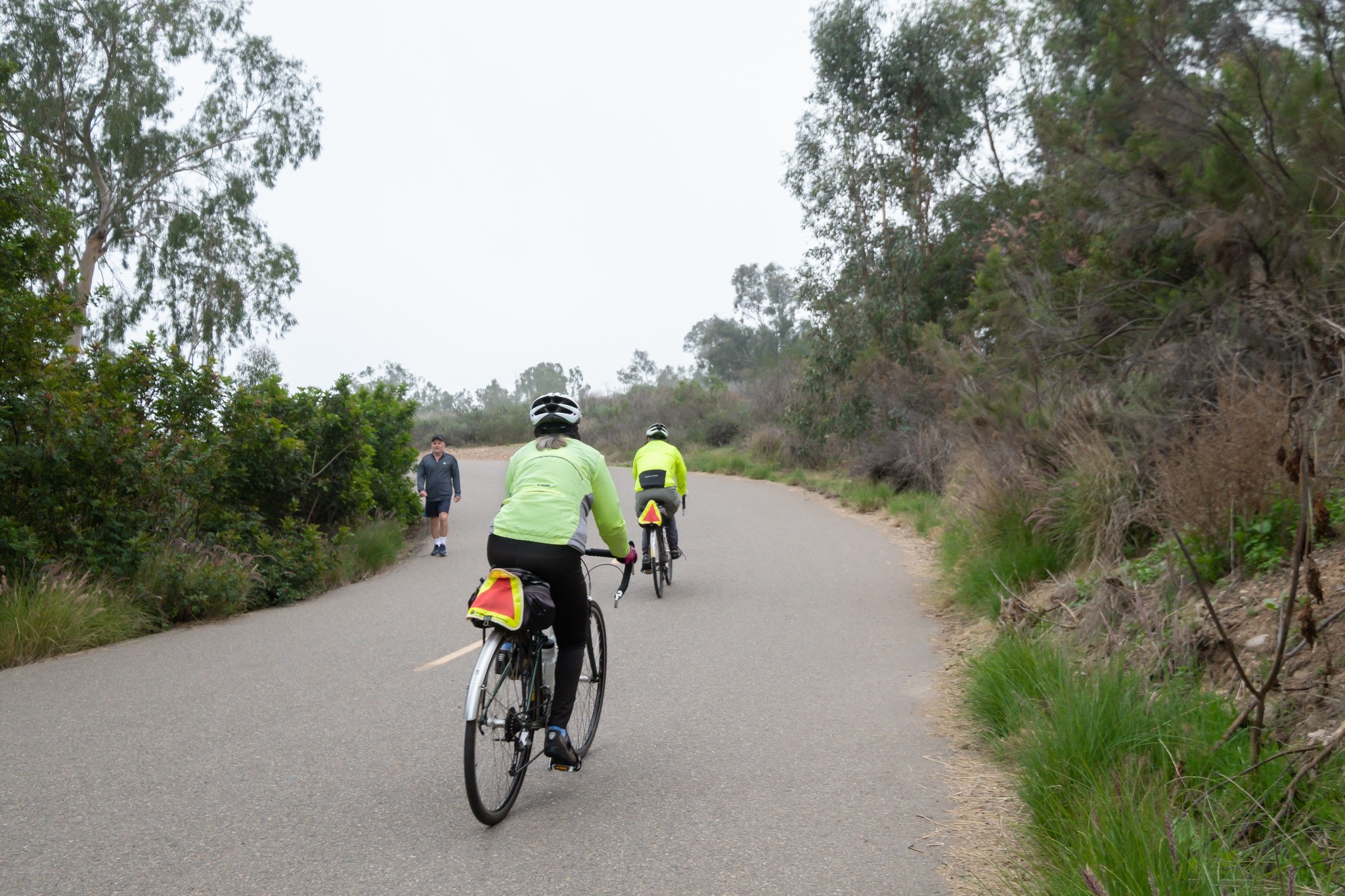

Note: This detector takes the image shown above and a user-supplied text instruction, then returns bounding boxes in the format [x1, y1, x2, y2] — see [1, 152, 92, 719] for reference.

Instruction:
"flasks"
[541, 639, 556, 695]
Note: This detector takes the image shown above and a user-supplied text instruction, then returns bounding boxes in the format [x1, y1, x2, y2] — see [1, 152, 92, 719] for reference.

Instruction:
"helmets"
[529, 392, 581, 425]
[645, 422, 668, 440]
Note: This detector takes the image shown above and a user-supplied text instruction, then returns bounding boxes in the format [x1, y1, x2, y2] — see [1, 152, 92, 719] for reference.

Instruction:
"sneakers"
[670, 549, 679, 560]
[430, 543, 439, 555]
[438, 544, 447, 556]
[642, 554, 651, 571]
[544, 728, 579, 767]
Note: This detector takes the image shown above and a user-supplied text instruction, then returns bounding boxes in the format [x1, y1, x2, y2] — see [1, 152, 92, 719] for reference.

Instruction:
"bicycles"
[459, 539, 639, 827]
[637, 495, 687, 598]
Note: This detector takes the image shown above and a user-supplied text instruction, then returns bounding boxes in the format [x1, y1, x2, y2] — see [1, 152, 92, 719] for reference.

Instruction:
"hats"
[431, 435, 444, 441]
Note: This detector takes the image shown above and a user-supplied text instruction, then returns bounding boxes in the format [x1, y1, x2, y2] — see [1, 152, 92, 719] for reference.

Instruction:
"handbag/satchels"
[468, 566, 556, 631]
[638, 499, 662, 527]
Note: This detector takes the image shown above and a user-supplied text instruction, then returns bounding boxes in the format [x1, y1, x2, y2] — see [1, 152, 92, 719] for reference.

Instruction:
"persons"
[631, 423, 686, 573]
[416, 434, 462, 556]
[487, 392, 637, 770]
